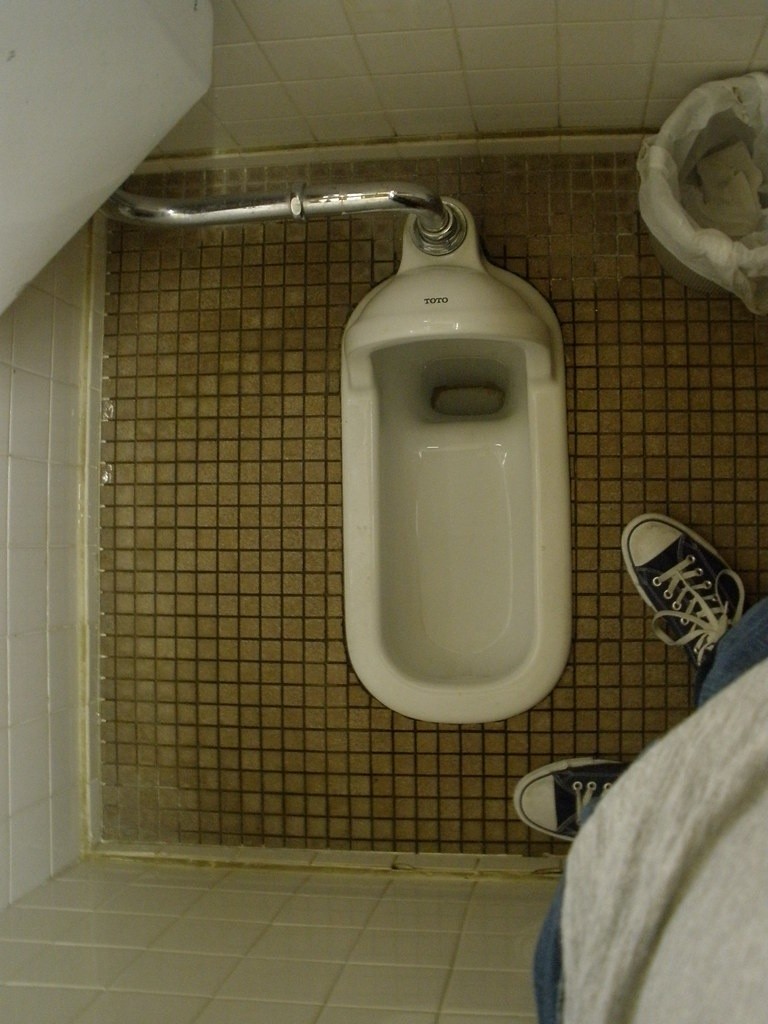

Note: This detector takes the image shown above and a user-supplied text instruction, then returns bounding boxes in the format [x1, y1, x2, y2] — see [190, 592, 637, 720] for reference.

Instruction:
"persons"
[511, 513, 768, 1024]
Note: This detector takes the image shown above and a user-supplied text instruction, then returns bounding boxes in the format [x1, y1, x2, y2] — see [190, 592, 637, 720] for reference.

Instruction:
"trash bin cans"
[639, 66, 768, 293]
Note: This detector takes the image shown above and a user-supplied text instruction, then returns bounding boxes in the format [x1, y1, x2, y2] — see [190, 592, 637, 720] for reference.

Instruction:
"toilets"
[338, 198, 574, 728]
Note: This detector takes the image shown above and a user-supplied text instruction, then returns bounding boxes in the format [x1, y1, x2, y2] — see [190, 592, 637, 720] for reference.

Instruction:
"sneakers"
[621, 513, 753, 669]
[512, 756, 630, 841]
